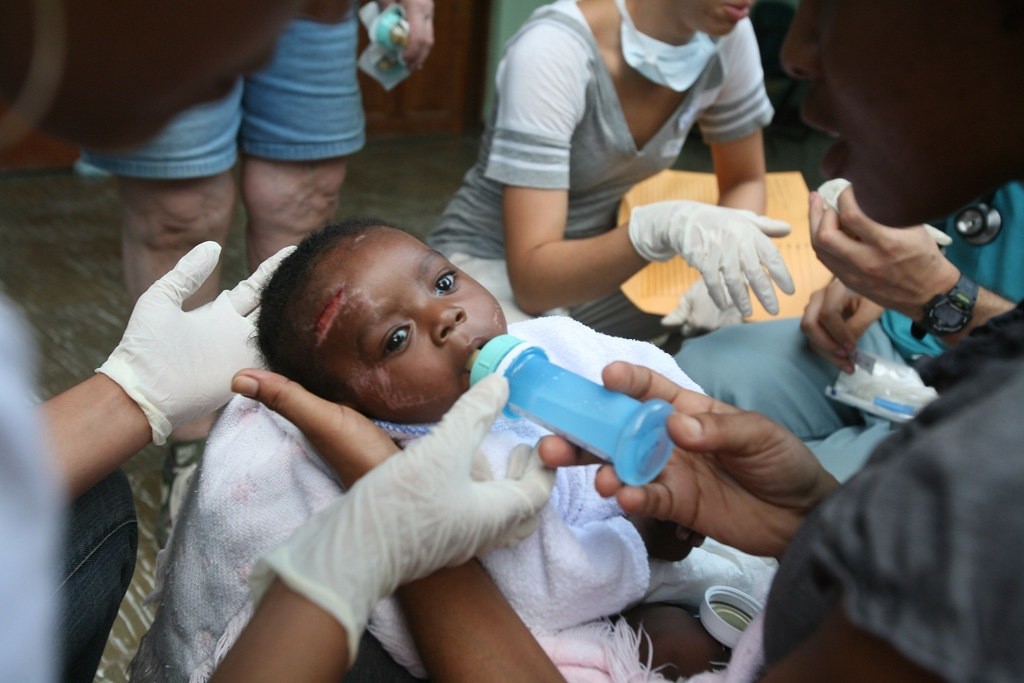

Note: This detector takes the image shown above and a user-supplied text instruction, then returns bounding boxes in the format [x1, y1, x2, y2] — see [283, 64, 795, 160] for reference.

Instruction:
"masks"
[614, 6, 723, 92]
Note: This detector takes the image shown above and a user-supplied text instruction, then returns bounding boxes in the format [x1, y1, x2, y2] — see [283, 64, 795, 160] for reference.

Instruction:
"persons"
[2, 218, 738, 683]
[1, 0, 773, 547]
[538, 0, 1024, 683]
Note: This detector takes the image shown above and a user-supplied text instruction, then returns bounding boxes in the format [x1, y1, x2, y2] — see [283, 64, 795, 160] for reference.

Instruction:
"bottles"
[464, 335, 677, 484]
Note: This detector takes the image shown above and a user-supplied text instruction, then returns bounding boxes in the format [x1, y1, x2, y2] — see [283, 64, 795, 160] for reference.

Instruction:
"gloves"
[97, 243, 311, 449]
[629, 197, 797, 338]
[254, 370, 559, 666]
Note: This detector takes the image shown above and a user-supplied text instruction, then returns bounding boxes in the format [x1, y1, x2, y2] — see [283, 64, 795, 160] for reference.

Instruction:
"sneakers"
[158, 437, 208, 556]
